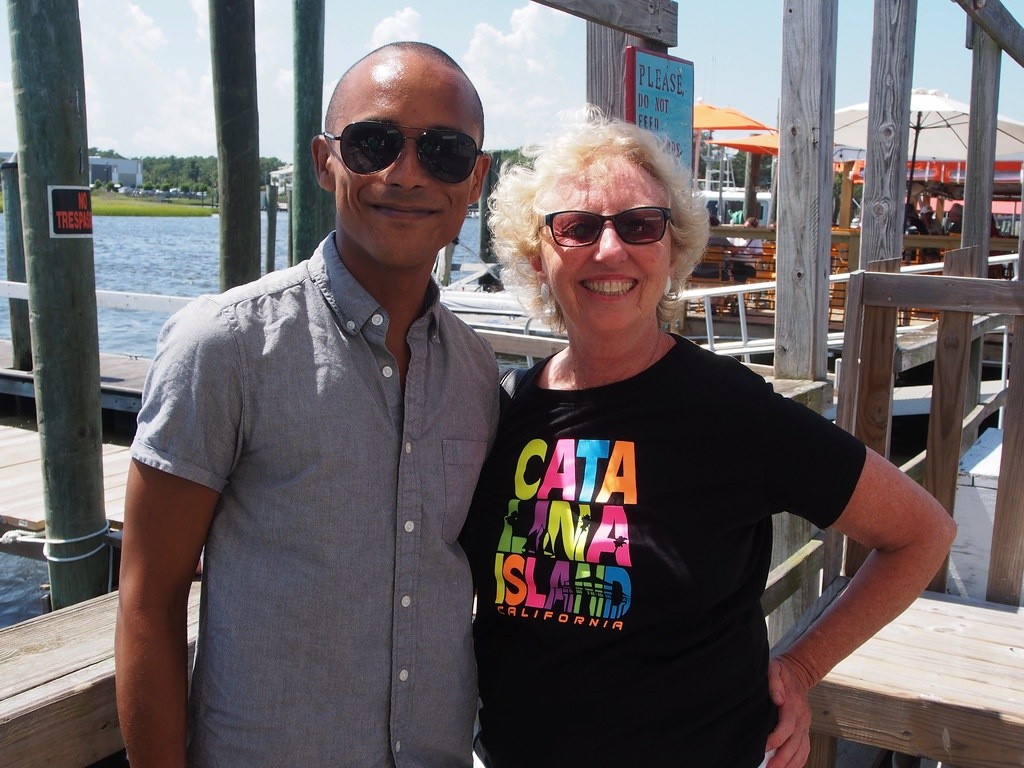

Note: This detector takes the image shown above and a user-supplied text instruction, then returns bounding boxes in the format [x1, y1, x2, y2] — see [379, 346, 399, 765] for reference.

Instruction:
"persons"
[690, 215, 767, 314]
[452, 101, 956, 767]
[903, 199, 1006, 279]
[115, 41, 500, 767]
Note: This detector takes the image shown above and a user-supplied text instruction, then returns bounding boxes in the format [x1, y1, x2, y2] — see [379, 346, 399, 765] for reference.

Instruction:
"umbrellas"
[692, 104, 775, 191]
[706, 130, 780, 157]
[833, 89, 1024, 228]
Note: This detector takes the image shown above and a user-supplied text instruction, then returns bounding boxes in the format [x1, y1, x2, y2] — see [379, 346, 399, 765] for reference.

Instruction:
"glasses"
[324, 121, 482, 184]
[546, 206, 675, 247]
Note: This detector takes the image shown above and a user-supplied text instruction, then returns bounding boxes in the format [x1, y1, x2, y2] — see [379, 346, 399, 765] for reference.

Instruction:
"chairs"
[685, 227, 1001, 325]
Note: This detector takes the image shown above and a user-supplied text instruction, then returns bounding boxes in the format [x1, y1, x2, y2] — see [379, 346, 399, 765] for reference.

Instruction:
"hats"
[920, 205, 934, 215]
